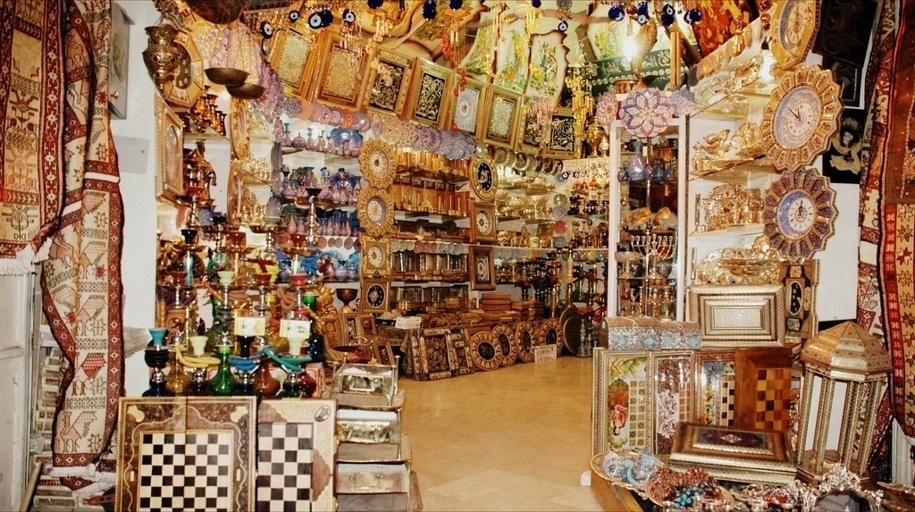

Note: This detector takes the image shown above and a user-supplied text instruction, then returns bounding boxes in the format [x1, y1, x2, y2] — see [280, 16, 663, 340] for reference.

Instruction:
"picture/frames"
[311, 31, 369, 109]
[480, 85, 521, 151]
[361, 49, 411, 114]
[600, 352, 650, 459]
[670, 427, 797, 468]
[256, 401, 337, 510]
[359, 315, 376, 341]
[115, 398, 257, 512]
[735, 346, 791, 431]
[651, 349, 695, 457]
[543, 109, 581, 160]
[376, 339, 395, 369]
[447, 75, 483, 136]
[698, 346, 737, 428]
[517, 100, 546, 153]
[343, 314, 361, 344]
[322, 319, 340, 347]
[402, 58, 454, 128]
[266, 22, 318, 99]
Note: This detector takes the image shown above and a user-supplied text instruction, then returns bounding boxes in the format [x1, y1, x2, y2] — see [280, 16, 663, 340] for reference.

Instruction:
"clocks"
[758, 67, 845, 171]
[466, 243, 497, 289]
[357, 187, 394, 235]
[764, 166, 836, 263]
[539, 319, 562, 355]
[471, 333, 502, 370]
[494, 325, 518, 365]
[472, 202, 497, 240]
[360, 140, 396, 187]
[156, 96, 185, 203]
[771, 0, 817, 65]
[359, 280, 388, 311]
[469, 155, 499, 201]
[360, 238, 389, 277]
[514, 322, 536, 362]
